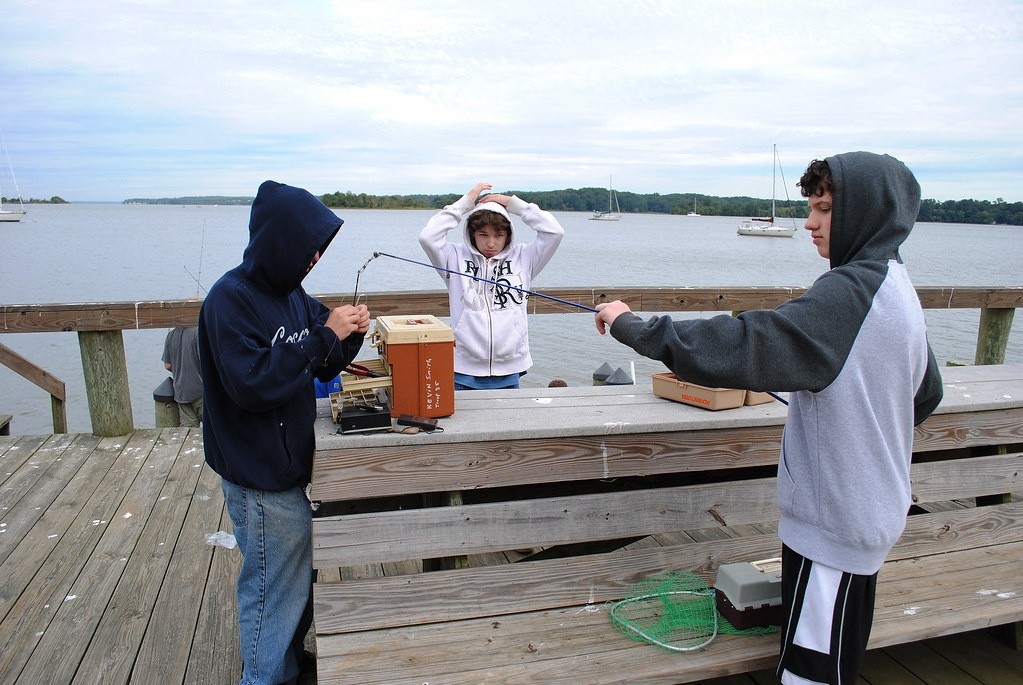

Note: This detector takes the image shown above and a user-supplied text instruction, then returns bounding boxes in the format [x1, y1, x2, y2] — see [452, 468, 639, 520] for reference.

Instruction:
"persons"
[198, 181, 372, 685]
[162, 327, 203, 428]
[593, 150, 944, 685]
[418, 182, 565, 388]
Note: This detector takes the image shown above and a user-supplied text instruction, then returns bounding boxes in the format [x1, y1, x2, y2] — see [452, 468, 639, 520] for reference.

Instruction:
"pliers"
[342, 363, 386, 378]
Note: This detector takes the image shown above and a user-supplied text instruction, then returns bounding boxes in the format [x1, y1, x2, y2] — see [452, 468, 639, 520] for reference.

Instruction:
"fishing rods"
[194, 212, 206, 301]
[181, 264, 208, 295]
[347, 252, 791, 410]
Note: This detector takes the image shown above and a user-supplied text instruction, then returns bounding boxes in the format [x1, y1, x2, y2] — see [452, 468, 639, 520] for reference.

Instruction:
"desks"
[301, 361, 1020, 445]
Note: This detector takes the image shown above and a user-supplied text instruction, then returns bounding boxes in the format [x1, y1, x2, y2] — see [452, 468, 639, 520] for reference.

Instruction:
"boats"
[0, 133, 26, 222]
[736, 143, 797, 237]
[587, 172, 622, 221]
[686, 196, 700, 217]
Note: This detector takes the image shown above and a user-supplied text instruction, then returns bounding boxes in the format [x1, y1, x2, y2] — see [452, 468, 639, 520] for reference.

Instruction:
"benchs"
[308, 403, 1022, 683]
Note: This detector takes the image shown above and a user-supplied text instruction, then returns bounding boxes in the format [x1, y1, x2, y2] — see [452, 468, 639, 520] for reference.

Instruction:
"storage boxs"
[368, 309, 457, 422]
[712, 554, 790, 629]
[648, 367, 778, 411]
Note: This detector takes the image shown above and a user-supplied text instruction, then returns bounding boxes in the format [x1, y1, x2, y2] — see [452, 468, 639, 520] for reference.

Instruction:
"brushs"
[374, 425, 420, 434]
